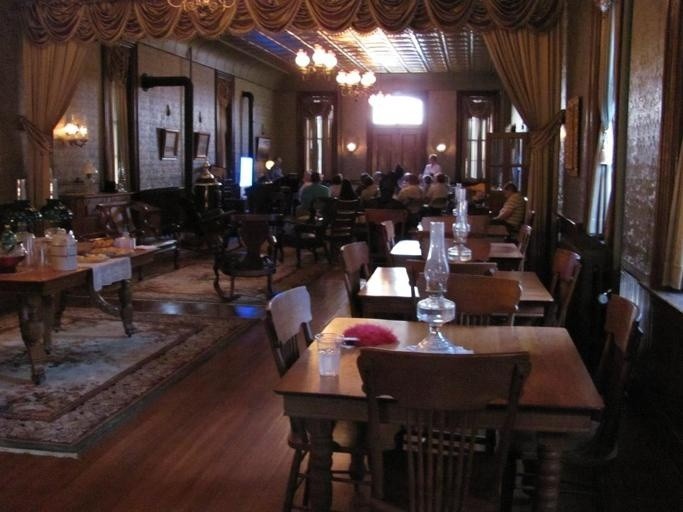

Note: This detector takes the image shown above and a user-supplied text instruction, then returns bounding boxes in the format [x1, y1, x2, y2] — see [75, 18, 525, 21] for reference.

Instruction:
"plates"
[77, 256, 107, 263]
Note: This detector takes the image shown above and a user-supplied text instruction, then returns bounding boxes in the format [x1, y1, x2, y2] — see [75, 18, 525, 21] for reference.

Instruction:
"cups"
[314, 331, 343, 376]
[114, 237, 136, 250]
[17, 225, 78, 272]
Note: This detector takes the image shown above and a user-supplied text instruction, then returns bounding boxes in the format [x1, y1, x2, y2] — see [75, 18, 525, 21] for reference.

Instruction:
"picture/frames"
[192, 131, 212, 160]
[159, 128, 177, 162]
[254, 136, 272, 163]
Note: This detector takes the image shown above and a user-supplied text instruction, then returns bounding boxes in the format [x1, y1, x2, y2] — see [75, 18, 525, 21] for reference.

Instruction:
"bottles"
[1, 224, 16, 255]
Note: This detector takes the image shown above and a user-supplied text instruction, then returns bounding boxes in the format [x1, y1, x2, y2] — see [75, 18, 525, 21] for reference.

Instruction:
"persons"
[250, 154, 451, 226]
[489, 182, 525, 235]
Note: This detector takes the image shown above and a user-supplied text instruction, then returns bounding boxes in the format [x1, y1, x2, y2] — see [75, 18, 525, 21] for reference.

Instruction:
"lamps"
[294, 42, 339, 83]
[82, 163, 97, 181]
[333, 66, 376, 98]
[62, 122, 90, 148]
